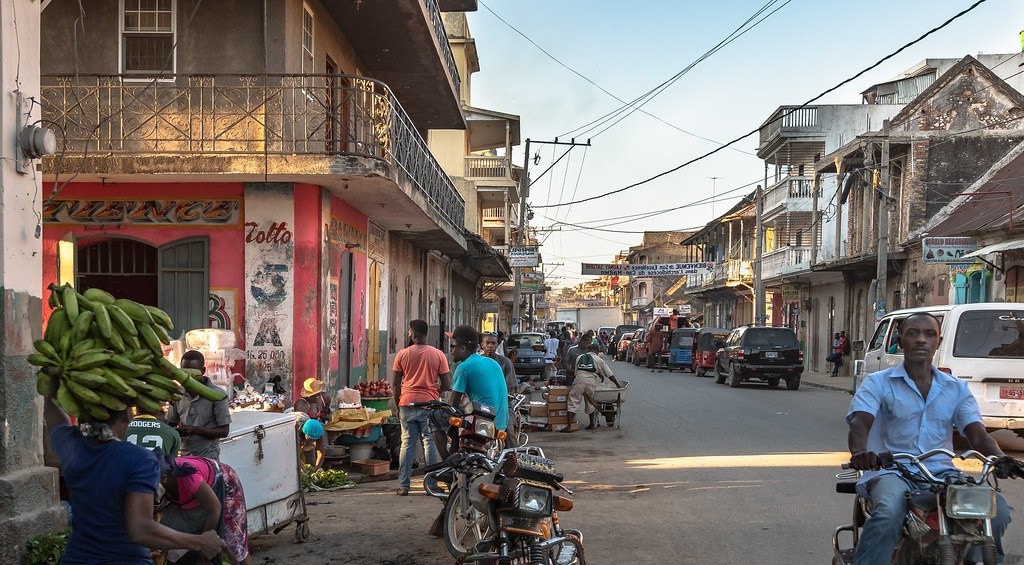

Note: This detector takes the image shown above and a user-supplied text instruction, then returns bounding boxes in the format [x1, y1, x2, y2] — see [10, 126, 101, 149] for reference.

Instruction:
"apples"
[354, 379, 394, 396]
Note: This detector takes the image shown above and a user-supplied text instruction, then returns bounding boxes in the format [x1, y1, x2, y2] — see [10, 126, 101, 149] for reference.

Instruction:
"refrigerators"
[219, 410, 304, 535]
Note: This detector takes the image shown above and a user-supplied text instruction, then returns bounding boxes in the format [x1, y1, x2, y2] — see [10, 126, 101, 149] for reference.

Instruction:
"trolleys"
[586, 379, 630, 429]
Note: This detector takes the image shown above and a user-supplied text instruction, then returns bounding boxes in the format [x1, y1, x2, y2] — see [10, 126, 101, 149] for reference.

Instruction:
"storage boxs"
[548, 385, 569, 396]
[547, 418, 568, 425]
[548, 411, 568, 417]
[546, 395, 567, 402]
[352, 459, 390, 475]
[547, 403, 567, 411]
[527, 416, 547, 424]
[529, 406, 548, 417]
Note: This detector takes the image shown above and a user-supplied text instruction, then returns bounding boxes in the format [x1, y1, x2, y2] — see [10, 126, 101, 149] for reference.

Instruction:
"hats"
[301, 377, 324, 397]
[301, 419, 323, 440]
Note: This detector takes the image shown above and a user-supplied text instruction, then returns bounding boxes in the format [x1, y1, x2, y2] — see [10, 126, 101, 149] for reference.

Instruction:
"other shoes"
[829, 373, 836, 377]
[658, 369, 663, 373]
[651, 369, 654, 372]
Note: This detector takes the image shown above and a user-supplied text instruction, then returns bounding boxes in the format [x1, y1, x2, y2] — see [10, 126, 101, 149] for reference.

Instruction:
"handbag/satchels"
[842, 337, 850, 355]
[577, 353, 596, 372]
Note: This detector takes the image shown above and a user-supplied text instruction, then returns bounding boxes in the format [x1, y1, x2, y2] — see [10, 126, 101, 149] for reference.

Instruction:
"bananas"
[299, 463, 352, 489]
[26, 282, 229, 421]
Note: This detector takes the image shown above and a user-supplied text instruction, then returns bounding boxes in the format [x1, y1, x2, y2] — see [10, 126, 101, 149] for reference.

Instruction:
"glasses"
[450, 343, 460, 348]
[482, 331, 498, 336]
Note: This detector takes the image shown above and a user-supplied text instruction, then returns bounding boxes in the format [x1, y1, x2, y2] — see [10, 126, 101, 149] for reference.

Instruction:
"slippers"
[585, 423, 595, 430]
[427, 487, 445, 495]
[560, 428, 574, 433]
[396, 488, 407, 496]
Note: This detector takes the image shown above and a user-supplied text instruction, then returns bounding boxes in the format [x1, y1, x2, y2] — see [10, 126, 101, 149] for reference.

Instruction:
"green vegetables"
[22, 526, 75, 565]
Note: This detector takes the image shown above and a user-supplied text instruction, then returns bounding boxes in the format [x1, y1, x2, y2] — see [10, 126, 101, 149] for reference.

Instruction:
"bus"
[546, 320, 576, 339]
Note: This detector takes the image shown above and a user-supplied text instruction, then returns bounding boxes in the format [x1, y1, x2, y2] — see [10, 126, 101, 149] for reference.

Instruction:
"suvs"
[713, 322, 804, 389]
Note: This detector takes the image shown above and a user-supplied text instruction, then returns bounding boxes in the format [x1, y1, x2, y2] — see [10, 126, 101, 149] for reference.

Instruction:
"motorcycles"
[832, 448, 1024, 565]
[404, 389, 587, 565]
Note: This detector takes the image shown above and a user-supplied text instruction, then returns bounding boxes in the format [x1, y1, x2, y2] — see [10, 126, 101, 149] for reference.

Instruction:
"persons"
[846, 313, 1024, 565]
[668, 309, 679, 330]
[825, 331, 848, 377]
[557, 327, 620, 384]
[287, 411, 325, 469]
[125, 405, 180, 459]
[479, 332, 518, 448]
[295, 378, 330, 421]
[560, 344, 622, 433]
[647, 324, 663, 372]
[429, 325, 509, 462]
[544, 331, 559, 382]
[687, 318, 701, 328]
[496, 331, 507, 357]
[43, 397, 226, 565]
[393, 319, 451, 496]
[151, 446, 255, 565]
[154, 351, 233, 462]
[432, 331, 459, 458]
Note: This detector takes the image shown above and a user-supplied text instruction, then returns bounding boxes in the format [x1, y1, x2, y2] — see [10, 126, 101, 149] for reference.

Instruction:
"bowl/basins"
[339, 426, 380, 442]
[361, 397, 389, 411]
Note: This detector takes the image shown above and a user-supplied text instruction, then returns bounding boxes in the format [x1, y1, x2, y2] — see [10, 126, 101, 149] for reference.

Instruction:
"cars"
[596, 325, 731, 377]
[503, 332, 548, 380]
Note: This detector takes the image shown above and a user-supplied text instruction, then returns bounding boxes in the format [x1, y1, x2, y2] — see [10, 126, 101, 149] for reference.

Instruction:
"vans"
[859, 303, 1024, 431]
